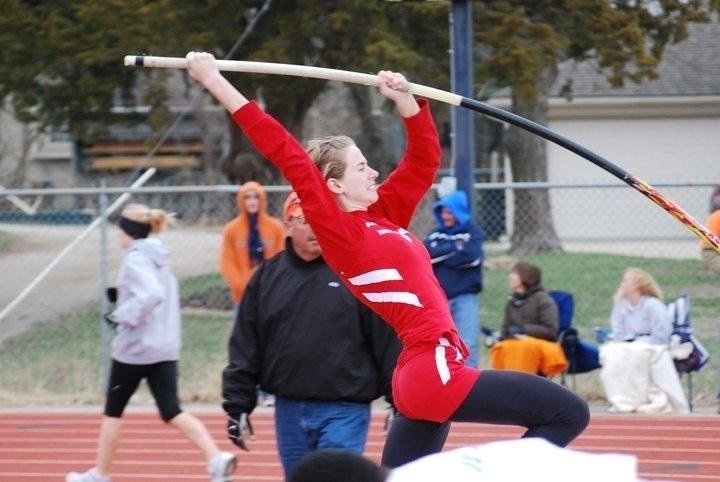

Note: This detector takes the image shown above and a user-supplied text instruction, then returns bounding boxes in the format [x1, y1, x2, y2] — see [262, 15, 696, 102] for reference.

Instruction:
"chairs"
[584, 291, 711, 415]
[478, 287, 601, 389]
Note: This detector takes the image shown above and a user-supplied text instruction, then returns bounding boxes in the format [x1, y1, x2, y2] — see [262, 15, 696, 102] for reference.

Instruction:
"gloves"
[228, 413, 255, 452]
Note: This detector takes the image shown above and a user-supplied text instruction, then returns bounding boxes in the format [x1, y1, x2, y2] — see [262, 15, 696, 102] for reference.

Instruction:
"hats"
[283, 191, 305, 226]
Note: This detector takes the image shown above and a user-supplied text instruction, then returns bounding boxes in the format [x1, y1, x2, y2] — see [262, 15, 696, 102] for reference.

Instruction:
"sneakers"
[207, 452, 237, 482]
[66, 467, 112, 482]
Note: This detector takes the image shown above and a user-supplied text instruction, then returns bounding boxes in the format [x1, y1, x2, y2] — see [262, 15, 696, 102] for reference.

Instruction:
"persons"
[420, 191, 488, 368]
[187, 52, 589, 469]
[699, 196, 720, 272]
[598, 265, 690, 414]
[285, 437, 637, 482]
[65, 203, 238, 482]
[222, 190, 404, 482]
[491, 260, 570, 376]
[222, 180, 285, 407]
